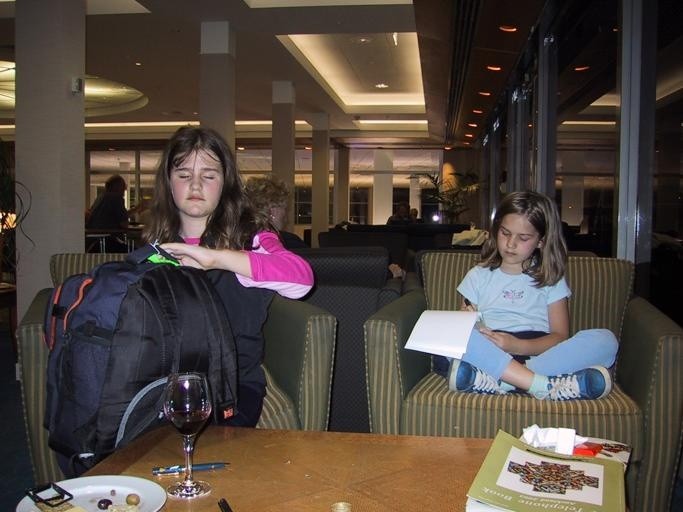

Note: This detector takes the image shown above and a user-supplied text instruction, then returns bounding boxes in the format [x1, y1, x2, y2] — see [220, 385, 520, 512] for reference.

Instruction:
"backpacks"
[42, 245, 237, 477]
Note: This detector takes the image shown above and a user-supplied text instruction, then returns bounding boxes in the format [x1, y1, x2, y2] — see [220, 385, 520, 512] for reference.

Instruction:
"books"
[465, 429, 626, 512]
[404, 310, 485, 360]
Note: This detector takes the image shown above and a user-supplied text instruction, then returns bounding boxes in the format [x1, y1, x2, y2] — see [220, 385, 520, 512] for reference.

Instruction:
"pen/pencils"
[152, 463, 231, 475]
[218, 497, 233, 512]
[464, 298, 474, 312]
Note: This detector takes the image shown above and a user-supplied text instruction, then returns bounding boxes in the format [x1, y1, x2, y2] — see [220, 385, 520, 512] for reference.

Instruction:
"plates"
[14, 475, 166, 511]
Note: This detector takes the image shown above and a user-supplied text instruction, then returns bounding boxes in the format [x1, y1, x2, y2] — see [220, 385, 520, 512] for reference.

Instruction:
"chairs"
[363, 248, 682, 510]
[14, 253, 336, 490]
[281, 246, 401, 433]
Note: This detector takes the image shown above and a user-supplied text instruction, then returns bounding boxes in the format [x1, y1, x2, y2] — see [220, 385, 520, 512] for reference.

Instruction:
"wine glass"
[161, 373, 212, 500]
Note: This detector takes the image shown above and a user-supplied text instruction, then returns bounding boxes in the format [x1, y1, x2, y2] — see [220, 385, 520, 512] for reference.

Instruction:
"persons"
[387, 201, 413, 225]
[136, 125, 315, 300]
[445, 190, 619, 401]
[242, 176, 309, 250]
[86, 176, 148, 253]
[410, 209, 424, 224]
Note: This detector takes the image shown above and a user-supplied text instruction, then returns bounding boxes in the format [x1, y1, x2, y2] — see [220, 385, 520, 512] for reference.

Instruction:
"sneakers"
[448, 358, 505, 396]
[537, 365, 613, 400]
